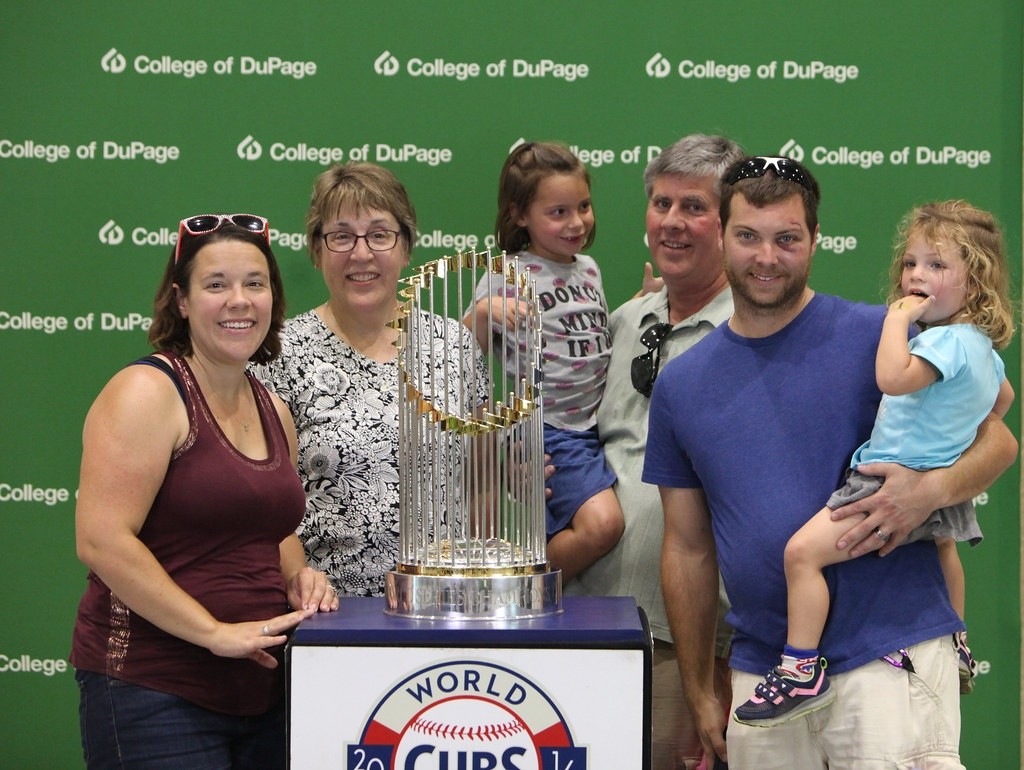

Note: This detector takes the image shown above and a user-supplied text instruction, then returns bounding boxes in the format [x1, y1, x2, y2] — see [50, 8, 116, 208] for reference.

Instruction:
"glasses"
[722, 156, 819, 207]
[316, 228, 403, 252]
[175, 214, 270, 264]
[631, 323, 674, 398]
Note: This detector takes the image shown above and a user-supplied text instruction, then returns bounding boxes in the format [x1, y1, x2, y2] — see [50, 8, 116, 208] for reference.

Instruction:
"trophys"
[383, 245, 564, 621]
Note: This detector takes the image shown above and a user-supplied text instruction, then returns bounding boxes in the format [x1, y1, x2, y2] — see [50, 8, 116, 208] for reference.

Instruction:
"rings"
[262, 625, 270, 636]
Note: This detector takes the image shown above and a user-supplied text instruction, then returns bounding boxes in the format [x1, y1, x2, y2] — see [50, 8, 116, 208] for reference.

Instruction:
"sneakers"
[733, 657, 836, 729]
[956, 639, 976, 697]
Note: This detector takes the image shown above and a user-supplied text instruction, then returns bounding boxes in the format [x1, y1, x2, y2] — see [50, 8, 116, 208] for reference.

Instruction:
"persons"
[66, 215, 341, 769]
[243, 159, 496, 596]
[639, 154, 1020, 770]
[461, 142, 663, 597]
[502, 134, 748, 770]
